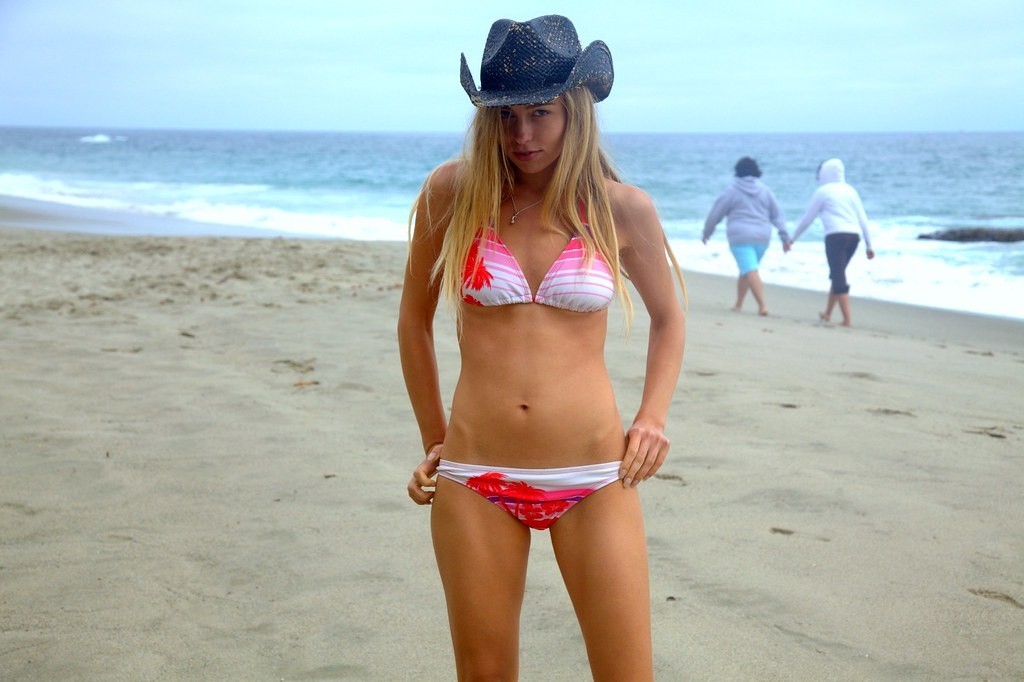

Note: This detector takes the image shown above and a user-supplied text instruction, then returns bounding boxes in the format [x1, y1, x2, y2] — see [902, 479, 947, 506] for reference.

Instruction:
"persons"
[701, 156, 790, 317]
[396, 14, 689, 682]
[791, 157, 875, 327]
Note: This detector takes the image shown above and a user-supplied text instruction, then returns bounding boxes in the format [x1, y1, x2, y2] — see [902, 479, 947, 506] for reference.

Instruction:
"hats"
[461, 15, 614, 108]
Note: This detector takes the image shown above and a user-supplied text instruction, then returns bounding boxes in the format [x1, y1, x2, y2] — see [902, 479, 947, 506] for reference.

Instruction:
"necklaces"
[509, 189, 544, 224]
